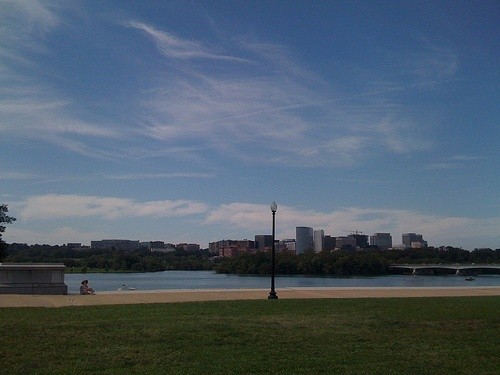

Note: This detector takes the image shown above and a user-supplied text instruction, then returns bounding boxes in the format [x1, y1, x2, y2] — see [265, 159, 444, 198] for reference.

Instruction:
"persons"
[80, 280, 95, 295]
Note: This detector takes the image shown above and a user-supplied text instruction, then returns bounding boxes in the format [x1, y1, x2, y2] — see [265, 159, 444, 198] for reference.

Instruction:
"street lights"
[267, 201, 281, 299]
